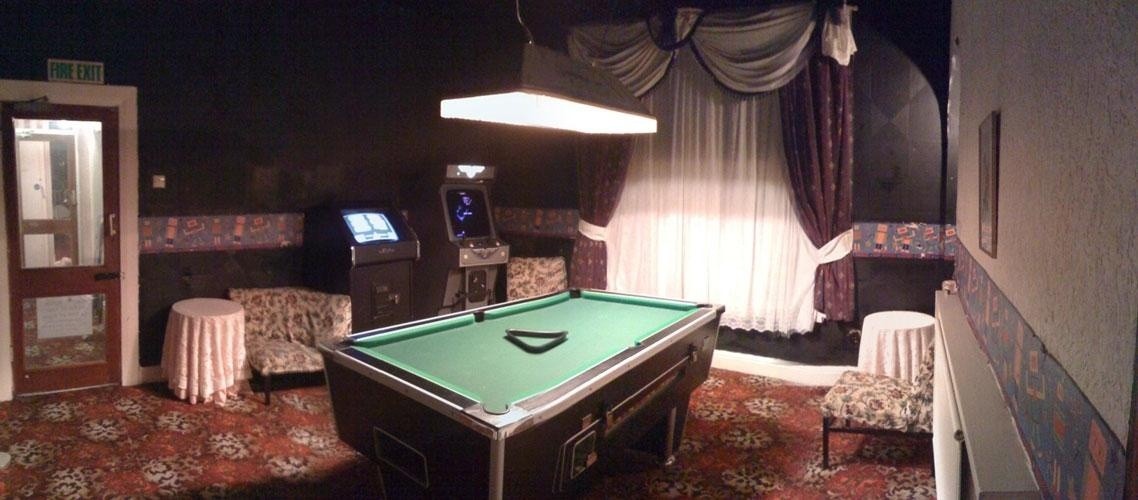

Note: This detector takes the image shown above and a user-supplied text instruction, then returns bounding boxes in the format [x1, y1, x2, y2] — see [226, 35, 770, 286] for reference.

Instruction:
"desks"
[314, 281, 727, 498]
[857, 309, 935, 380]
[169, 299, 245, 400]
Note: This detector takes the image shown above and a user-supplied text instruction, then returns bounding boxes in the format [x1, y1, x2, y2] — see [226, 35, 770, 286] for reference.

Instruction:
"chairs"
[818, 347, 935, 470]
[228, 284, 352, 406]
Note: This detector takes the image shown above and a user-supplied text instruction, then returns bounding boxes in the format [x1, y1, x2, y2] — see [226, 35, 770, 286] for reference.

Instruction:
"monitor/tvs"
[343, 212, 400, 244]
[446, 189, 490, 239]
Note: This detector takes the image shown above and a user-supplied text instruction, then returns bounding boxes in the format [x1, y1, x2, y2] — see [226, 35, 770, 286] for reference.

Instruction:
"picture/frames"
[975, 111, 999, 259]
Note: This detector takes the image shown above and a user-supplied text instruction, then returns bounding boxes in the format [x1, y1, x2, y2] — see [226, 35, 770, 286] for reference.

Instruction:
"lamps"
[437, 0, 661, 141]
